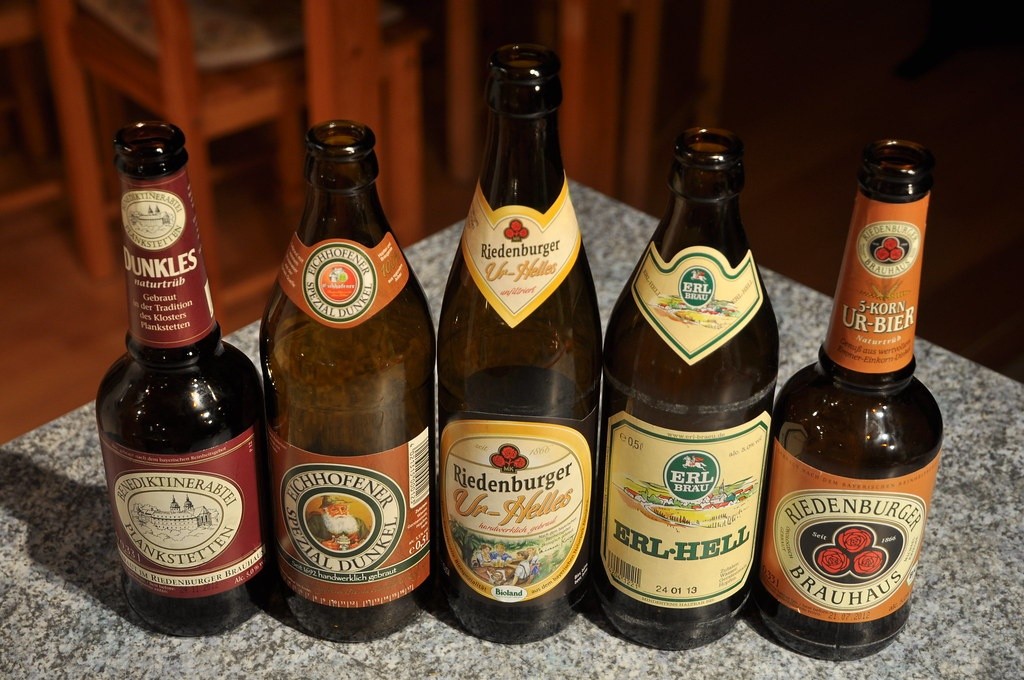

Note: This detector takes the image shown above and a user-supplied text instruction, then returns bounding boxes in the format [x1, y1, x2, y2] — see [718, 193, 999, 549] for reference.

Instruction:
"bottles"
[595, 126, 780, 652]
[258, 119, 439, 644]
[96, 122, 277, 637]
[753, 140, 945, 664]
[437, 43, 603, 643]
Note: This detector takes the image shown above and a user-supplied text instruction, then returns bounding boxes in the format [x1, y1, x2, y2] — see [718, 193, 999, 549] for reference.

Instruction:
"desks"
[0, 179, 1024, 680]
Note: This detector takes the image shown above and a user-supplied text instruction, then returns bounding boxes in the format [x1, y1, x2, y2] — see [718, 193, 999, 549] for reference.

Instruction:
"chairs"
[0, 0, 735, 317]
